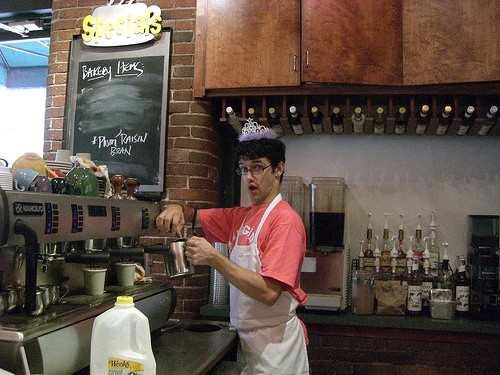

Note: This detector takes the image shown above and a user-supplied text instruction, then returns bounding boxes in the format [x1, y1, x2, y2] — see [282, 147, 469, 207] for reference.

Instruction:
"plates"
[45, 161, 73, 170]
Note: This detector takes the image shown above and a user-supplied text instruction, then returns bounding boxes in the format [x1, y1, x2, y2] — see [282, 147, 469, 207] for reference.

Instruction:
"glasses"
[236, 163, 274, 176]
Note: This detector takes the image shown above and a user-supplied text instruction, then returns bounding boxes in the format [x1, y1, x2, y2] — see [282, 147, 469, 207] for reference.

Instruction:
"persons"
[156, 138, 312, 375]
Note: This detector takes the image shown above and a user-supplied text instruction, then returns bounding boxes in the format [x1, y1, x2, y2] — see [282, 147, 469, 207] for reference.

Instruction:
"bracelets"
[179, 204, 185, 212]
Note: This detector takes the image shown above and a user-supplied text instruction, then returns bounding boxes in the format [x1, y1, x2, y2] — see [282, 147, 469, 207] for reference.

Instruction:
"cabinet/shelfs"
[193, 0, 500, 97]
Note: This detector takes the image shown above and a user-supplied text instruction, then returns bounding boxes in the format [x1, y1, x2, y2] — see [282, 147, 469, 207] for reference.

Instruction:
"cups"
[0, 158, 8, 167]
[163, 239, 195, 280]
[209, 241, 229, 305]
[115, 263, 136, 286]
[13, 164, 39, 192]
[0, 285, 69, 316]
[84, 268, 107, 295]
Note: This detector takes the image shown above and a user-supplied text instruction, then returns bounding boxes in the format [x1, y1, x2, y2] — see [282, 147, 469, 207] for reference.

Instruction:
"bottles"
[331, 107, 366, 134]
[289, 106, 324, 135]
[352, 210, 407, 313]
[407, 214, 423, 316]
[441, 242, 469, 316]
[374, 107, 407, 135]
[248, 108, 284, 138]
[414, 105, 498, 136]
[226, 106, 243, 139]
[424, 211, 440, 307]
[90, 296, 156, 375]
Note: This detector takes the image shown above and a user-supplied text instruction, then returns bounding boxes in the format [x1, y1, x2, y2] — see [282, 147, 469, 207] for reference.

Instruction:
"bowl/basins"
[55, 149, 91, 163]
[12, 153, 47, 176]
[0, 166, 13, 190]
[51, 177, 71, 195]
[28, 176, 52, 193]
[67, 167, 100, 197]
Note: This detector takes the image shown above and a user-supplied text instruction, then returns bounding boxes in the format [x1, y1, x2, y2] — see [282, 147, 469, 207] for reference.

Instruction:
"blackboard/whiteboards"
[61, 27, 174, 198]
